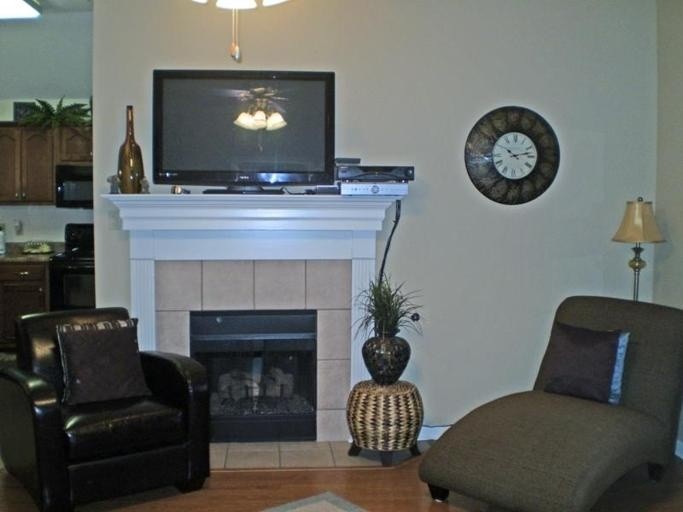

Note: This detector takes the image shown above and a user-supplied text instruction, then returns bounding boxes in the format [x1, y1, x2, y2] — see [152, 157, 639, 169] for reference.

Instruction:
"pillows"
[534, 320, 633, 408]
[52, 315, 152, 408]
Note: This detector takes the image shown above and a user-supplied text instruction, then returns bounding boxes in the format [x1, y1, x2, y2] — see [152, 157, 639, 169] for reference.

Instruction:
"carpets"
[256, 487, 370, 512]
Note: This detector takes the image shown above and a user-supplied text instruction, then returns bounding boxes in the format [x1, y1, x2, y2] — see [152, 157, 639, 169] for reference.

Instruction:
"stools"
[343, 380, 424, 469]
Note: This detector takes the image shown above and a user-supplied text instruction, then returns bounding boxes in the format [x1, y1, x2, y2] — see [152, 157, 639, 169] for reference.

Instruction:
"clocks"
[461, 104, 561, 207]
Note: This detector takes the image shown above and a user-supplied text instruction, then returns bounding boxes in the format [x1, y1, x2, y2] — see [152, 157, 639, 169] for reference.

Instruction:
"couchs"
[416, 292, 683, 512]
[0, 305, 211, 512]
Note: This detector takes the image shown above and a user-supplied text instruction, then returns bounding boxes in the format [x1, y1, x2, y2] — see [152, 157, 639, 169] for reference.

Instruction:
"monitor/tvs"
[153, 70, 335, 194]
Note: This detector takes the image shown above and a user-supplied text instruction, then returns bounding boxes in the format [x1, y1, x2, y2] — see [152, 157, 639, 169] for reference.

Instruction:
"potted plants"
[350, 273, 428, 384]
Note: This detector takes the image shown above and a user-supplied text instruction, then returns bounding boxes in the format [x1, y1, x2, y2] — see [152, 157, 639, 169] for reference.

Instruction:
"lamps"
[610, 195, 669, 303]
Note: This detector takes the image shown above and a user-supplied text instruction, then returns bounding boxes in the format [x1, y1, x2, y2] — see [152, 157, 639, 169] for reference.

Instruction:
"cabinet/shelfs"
[0, 119, 93, 207]
[0, 249, 64, 355]
